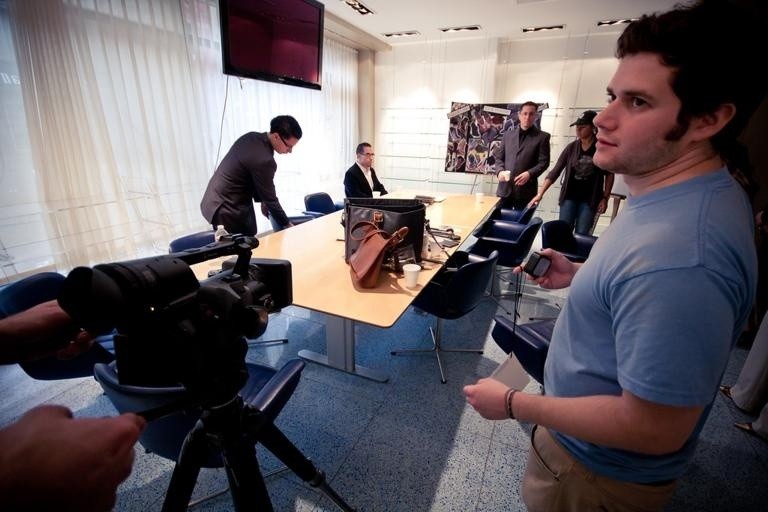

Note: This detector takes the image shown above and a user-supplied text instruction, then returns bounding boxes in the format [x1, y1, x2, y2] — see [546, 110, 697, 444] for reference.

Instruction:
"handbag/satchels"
[342, 197, 426, 265]
[348, 221, 409, 289]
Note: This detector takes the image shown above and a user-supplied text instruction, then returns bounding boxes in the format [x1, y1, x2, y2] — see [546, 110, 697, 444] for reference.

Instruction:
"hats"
[570, 110, 597, 127]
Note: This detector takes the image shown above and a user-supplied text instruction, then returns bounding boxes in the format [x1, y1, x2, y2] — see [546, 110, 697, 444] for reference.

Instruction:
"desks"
[187, 191, 505, 382]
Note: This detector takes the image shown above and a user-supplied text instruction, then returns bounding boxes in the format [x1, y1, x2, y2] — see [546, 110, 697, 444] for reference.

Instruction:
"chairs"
[168, 193, 345, 254]
[393, 203, 597, 384]
[93, 348, 318, 506]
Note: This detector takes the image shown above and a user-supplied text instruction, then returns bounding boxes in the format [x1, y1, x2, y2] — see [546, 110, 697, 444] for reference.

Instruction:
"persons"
[460, 5, 760, 512]
[492, 97, 552, 209]
[198, 111, 304, 243]
[0, 297, 148, 511]
[526, 107, 617, 240]
[342, 140, 388, 209]
[717, 306, 768, 441]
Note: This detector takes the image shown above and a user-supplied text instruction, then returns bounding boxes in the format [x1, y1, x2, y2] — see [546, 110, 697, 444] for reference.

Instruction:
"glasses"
[360, 152, 375, 157]
[279, 135, 293, 148]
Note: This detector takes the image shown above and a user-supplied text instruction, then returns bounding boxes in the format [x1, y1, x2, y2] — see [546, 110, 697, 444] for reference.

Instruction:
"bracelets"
[504, 387, 521, 422]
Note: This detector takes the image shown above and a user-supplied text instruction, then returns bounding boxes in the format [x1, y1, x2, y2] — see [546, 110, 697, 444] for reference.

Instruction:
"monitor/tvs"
[219, 0, 326, 92]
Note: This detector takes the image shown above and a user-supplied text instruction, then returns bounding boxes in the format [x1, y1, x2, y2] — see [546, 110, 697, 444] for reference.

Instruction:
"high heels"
[718, 385, 733, 398]
[734, 422, 754, 434]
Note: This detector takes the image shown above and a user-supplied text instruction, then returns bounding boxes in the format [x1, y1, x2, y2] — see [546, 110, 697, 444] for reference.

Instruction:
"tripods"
[160, 394, 355, 512]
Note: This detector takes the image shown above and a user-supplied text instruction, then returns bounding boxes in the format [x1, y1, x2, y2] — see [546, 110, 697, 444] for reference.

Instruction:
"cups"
[475, 192, 484, 204]
[503, 170, 512, 182]
[372, 191, 381, 199]
[429, 236, 444, 257]
[402, 263, 421, 289]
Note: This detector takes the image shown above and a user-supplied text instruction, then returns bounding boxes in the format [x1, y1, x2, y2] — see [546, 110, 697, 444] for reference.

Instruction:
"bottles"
[421, 228, 429, 258]
[214, 224, 230, 242]
